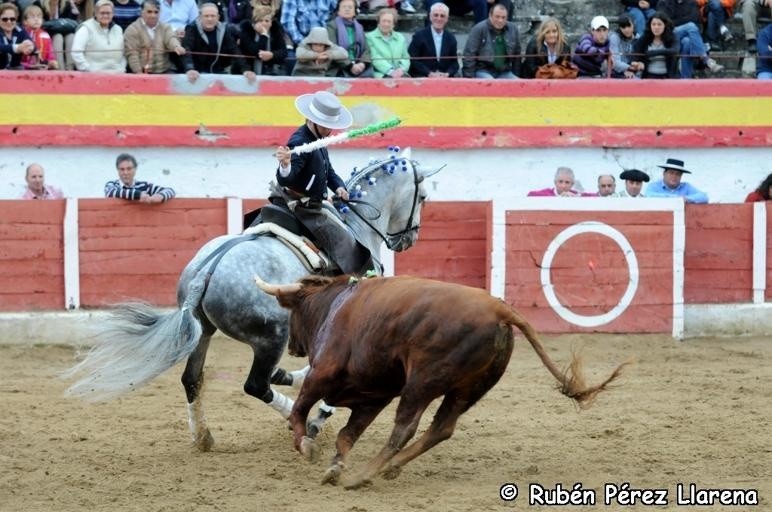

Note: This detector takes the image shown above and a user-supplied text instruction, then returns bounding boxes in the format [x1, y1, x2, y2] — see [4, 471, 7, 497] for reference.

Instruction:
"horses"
[58, 146, 448, 452]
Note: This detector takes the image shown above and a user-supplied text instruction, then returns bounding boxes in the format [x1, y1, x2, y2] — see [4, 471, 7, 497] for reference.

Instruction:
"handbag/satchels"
[535, 63, 578, 79]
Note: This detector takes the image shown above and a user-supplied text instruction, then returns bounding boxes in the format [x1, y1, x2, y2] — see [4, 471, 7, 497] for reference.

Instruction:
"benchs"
[511, 15, 550, 23]
[356, 12, 427, 21]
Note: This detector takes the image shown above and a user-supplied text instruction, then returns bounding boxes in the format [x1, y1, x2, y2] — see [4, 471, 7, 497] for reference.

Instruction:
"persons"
[104, 153, 175, 205]
[744, 172, 772, 203]
[527, 158, 709, 204]
[19, 163, 64, 200]
[275, 90, 374, 275]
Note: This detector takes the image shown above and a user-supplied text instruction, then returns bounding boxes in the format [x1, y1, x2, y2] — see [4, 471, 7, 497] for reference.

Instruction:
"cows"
[254, 261, 634, 490]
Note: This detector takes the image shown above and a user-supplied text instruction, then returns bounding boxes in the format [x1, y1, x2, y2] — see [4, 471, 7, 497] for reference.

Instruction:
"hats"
[305, 26, 332, 48]
[656, 158, 693, 176]
[619, 169, 650, 183]
[294, 91, 353, 129]
[590, 15, 610, 31]
